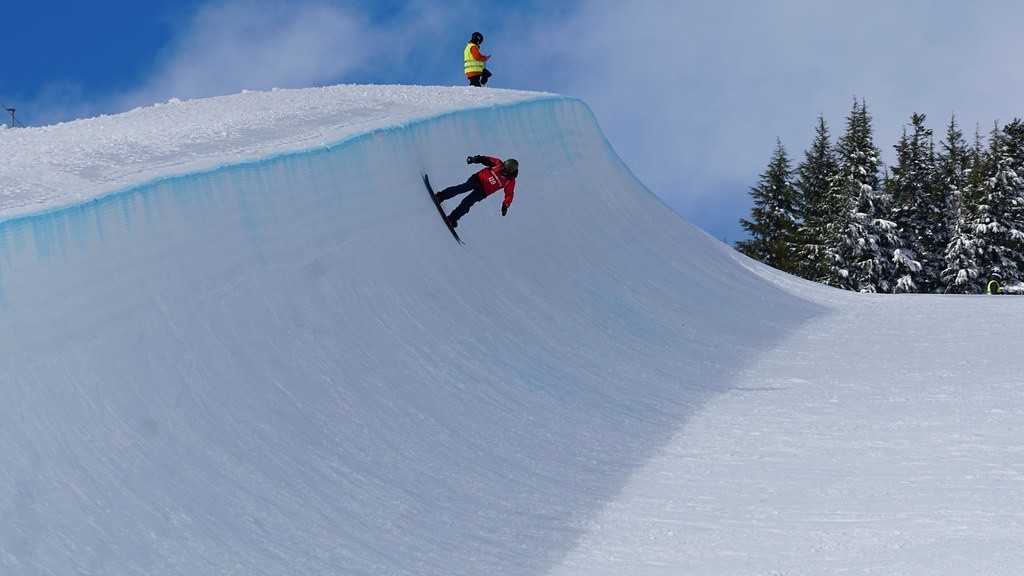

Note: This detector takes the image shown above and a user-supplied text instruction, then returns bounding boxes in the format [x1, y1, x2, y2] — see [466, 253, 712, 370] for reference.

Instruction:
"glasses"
[479, 41, 482, 44]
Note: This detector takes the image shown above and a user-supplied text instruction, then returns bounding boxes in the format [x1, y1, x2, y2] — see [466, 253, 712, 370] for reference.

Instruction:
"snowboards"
[424, 173, 467, 246]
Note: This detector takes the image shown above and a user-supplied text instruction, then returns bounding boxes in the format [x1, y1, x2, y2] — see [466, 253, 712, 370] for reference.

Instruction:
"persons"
[436, 155, 519, 227]
[464, 31, 492, 87]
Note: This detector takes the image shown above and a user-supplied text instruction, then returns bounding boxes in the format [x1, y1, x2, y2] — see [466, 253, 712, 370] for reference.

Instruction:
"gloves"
[501, 205, 507, 216]
[467, 156, 475, 164]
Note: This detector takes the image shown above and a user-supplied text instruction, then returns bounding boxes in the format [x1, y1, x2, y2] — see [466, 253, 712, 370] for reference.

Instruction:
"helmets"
[504, 159, 518, 173]
[472, 32, 483, 44]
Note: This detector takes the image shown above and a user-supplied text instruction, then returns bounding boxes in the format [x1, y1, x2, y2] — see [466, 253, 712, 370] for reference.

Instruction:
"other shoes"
[435, 194, 441, 204]
[447, 216, 457, 227]
